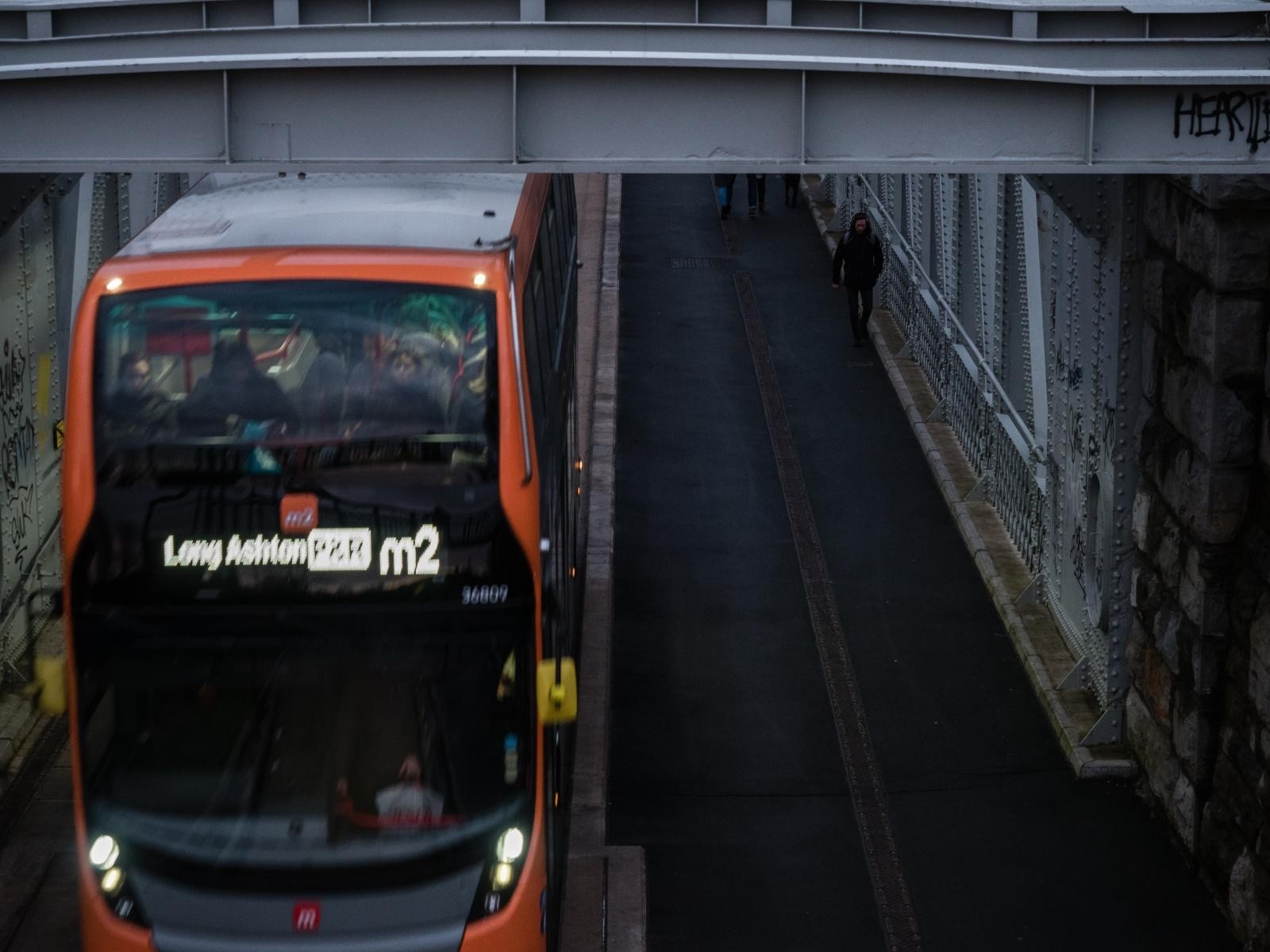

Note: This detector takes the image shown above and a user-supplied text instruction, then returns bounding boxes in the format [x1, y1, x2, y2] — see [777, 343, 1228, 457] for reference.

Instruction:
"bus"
[24, 172, 584, 951]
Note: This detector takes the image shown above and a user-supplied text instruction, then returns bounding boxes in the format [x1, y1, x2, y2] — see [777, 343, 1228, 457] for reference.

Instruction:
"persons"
[344, 296, 486, 436]
[747, 173, 767, 217]
[832, 213, 884, 347]
[101, 352, 180, 454]
[178, 336, 301, 438]
[782, 174, 801, 208]
[714, 173, 736, 220]
[334, 642, 425, 815]
